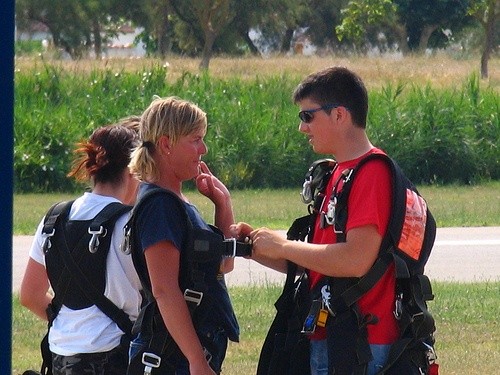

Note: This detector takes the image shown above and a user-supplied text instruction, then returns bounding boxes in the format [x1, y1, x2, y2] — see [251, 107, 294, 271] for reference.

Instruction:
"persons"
[227, 65, 439, 374]
[123, 96, 240, 375]
[19, 115, 143, 374]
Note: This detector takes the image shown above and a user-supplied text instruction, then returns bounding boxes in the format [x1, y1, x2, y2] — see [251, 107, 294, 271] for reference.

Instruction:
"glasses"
[299, 103, 347, 124]
